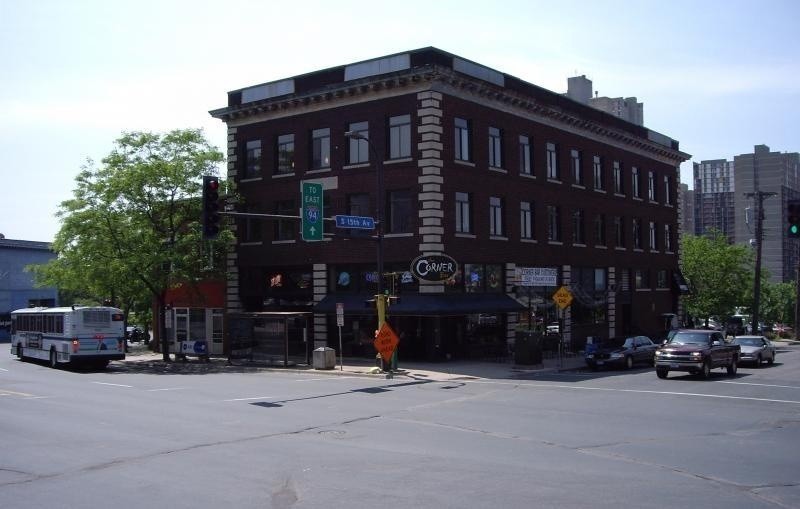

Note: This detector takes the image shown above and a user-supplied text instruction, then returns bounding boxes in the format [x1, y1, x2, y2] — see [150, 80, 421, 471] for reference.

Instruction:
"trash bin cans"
[585, 335, 601, 354]
[312, 346, 335, 370]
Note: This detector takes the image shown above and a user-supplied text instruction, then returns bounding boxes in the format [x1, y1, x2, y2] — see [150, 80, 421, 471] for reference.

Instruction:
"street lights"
[344, 131, 393, 373]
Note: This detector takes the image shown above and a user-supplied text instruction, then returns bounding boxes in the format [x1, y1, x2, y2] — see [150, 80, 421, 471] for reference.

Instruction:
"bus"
[9, 303, 126, 372]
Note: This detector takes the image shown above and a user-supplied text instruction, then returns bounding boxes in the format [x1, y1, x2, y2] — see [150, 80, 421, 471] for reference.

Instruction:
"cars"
[585, 306, 796, 380]
[126, 327, 141, 338]
[545, 321, 561, 336]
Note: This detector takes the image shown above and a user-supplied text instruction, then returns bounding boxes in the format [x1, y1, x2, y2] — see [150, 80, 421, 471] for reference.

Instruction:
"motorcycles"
[129, 330, 141, 343]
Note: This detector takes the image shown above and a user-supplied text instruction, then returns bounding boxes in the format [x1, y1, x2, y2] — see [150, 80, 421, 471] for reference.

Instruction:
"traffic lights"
[786, 202, 800, 239]
[203, 175, 220, 238]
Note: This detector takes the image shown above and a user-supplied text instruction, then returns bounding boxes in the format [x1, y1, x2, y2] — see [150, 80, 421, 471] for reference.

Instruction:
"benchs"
[173, 340, 211, 363]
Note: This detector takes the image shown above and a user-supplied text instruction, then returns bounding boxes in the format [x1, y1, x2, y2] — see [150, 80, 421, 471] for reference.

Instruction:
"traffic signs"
[302, 183, 324, 241]
